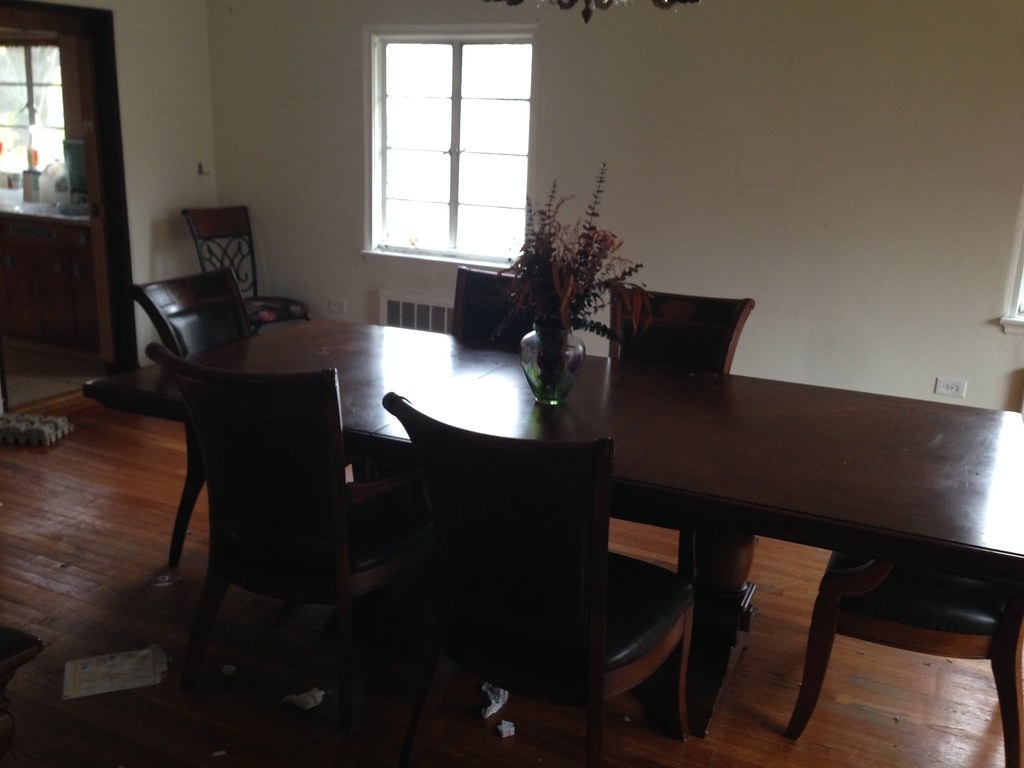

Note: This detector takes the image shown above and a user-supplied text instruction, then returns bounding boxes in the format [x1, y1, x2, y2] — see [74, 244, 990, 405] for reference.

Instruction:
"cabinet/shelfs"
[0, 212, 101, 355]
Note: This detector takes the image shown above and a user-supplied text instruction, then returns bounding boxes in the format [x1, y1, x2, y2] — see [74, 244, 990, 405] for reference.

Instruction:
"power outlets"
[934, 377, 968, 399]
[329, 298, 346, 313]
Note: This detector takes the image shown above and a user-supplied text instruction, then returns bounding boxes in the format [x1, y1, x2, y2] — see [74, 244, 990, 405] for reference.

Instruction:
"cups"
[23, 171, 40, 202]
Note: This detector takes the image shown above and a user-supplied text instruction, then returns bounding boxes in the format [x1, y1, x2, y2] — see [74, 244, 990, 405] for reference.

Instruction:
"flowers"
[491, 163, 647, 346]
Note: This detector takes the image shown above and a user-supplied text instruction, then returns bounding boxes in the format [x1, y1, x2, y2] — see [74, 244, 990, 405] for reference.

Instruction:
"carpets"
[0, 337, 97, 410]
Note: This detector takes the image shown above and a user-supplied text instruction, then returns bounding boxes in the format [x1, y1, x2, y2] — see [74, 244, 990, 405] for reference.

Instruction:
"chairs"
[382, 392, 692, 768]
[144, 342, 434, 741]
[130, 268, 248, 571]
[785, 542, 1024, 768]
[181, 204, 312, 334]
[449, 267, 535, 350]
[608, 285, 754, 583]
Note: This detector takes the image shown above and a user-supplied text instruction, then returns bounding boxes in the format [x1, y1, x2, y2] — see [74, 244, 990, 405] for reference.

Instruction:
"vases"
[521, 320, 586, 407]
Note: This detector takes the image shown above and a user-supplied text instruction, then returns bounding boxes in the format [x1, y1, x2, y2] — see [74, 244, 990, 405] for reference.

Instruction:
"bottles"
[38, 159, 71, 211]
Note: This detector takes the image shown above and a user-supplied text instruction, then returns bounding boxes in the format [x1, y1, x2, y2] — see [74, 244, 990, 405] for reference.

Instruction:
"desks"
[83, 317, 1024, 741]
